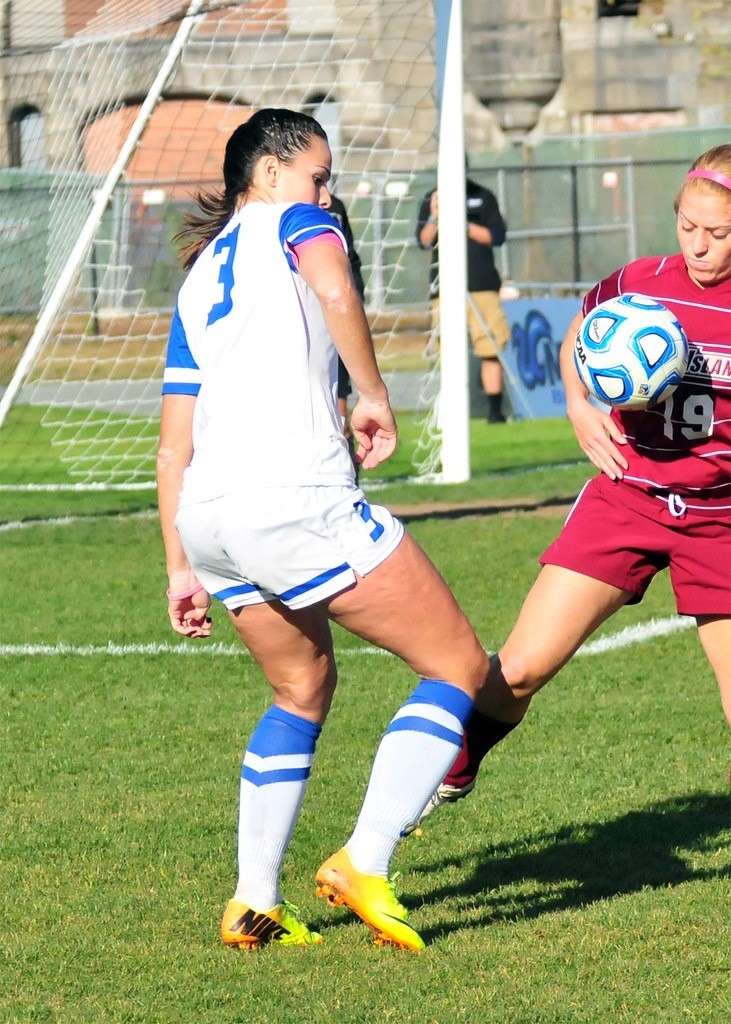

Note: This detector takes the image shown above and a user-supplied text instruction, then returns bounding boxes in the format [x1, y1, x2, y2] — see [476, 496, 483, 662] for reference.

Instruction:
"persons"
[154, 108, 490, 961]
[416, 147, 514, 426]
[393, 144, 729, 830]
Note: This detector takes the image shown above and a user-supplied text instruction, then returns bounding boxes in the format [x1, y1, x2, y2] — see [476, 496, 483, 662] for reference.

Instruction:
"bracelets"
[428, 217, 438, 228]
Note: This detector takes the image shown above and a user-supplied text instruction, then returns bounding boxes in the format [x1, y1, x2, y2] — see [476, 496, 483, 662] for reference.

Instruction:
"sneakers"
[392, 772, 479, 843]
[315, 850, 427, 959]
[218, 896, 325, 955]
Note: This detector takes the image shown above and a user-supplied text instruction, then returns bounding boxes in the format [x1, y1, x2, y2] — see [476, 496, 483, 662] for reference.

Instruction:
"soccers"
[572, 290, 691, 413]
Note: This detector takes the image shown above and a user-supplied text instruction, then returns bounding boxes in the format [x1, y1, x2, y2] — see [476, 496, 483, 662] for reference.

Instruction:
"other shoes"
[487, 410, 510, 426]
